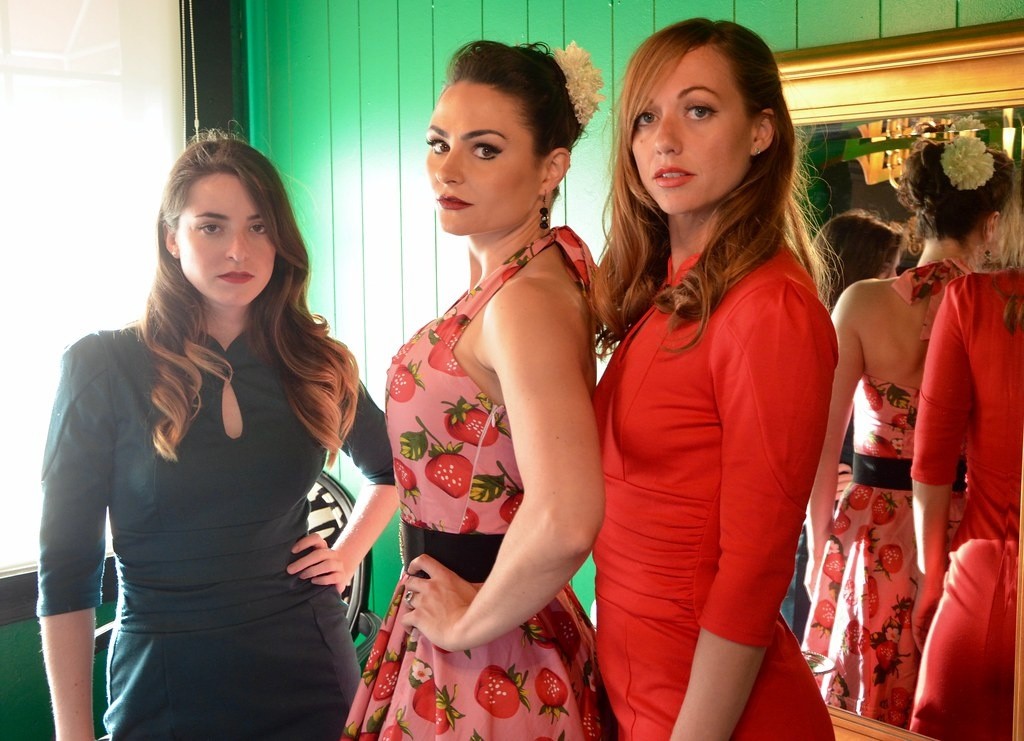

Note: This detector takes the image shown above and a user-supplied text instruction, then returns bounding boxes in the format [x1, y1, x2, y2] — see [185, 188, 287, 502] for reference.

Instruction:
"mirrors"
[772, 19, 1024, 741]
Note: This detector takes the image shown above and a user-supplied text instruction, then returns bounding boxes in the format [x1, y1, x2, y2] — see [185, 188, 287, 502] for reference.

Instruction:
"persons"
[347, 42, 605, 741]
[592, 18, 841, 741]
[35, 139, 397, 741]
[791, 130, 1022, 741]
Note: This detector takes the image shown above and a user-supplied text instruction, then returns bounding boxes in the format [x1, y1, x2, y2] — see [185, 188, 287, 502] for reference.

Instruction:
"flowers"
[553, 40, 606, 124]
[940, 136, 996, 190]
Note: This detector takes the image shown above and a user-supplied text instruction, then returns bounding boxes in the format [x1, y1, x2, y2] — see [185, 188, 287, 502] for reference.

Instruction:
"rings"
[404, 591, 415, 609]
